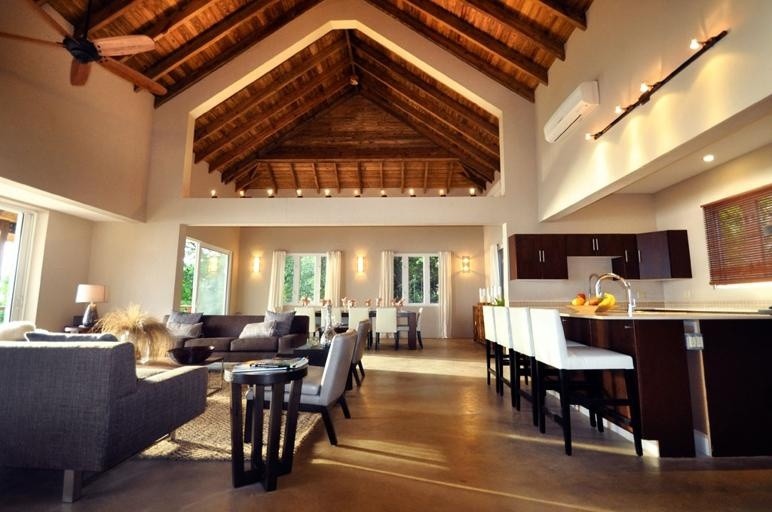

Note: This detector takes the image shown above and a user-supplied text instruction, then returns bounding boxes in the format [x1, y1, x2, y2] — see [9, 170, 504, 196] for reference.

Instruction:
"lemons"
[571, 297, 590, 306]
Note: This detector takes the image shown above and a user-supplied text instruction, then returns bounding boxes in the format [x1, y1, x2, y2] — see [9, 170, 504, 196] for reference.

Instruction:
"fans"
[0, 0, 168, 97]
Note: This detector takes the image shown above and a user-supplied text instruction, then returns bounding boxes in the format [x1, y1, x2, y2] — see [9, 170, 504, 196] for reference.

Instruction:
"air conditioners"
[543, 80, 599, 144]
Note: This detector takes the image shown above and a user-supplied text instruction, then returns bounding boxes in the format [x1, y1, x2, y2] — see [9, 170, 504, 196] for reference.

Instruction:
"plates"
[565, 304, 620, 314]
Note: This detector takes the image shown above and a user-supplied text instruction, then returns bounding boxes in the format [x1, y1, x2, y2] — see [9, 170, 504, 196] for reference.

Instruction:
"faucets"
[595, 273, 631, 298]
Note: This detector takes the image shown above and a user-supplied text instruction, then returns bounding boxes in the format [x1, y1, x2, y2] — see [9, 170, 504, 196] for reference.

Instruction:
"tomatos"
[576, 293, 585, 299]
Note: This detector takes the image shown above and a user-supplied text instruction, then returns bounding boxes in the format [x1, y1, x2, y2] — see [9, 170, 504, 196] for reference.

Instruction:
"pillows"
[168, 313, 203, 323]
[238, 320, 276, 338]
[167, 322, 203, 339]
[264, 310, 296, 336]
[24, 332, 117, 341]
[0, 321, 34, 341]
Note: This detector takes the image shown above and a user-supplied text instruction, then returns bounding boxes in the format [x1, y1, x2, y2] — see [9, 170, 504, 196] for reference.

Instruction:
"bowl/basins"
[167, 345, 216, 366]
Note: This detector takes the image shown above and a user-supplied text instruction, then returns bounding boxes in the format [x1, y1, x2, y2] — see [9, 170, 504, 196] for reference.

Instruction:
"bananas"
[596, 293, 615, 311]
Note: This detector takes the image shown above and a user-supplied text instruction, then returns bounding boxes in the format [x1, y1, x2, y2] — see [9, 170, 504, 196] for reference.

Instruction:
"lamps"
[75, 284, 105, 326]
[586, 31, 727, 141]
[210, 187, 477, 198]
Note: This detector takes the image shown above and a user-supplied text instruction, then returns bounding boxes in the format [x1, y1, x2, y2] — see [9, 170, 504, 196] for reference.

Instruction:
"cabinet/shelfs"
[509, 234, 568, 279]
[636, 230, 692, 280]
[567, 234, 623, 256]
[611, 234, 640, 280]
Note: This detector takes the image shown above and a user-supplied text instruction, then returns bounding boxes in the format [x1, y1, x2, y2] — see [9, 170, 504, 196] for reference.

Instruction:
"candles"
[479, 285, 502, 304]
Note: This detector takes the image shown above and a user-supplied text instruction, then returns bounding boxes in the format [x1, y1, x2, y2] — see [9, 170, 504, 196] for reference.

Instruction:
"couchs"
[163, 315, 309, 362]
[0, 341, 208, 503]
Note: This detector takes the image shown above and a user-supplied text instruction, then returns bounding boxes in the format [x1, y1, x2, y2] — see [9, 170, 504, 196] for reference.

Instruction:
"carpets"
[133, 362, 323, 462]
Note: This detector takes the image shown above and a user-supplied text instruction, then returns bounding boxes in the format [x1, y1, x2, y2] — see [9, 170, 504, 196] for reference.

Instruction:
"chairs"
[482, 305, 643, 458]
[294, 307, 423, 351]
[350, 320, 370, 387]
[244, 328, 358, 446]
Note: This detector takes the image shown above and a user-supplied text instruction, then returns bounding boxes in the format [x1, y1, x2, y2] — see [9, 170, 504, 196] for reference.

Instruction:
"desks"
[224, 360, 308, 492]
[294, 344, 353, 390]
[473, 305, 484, 344]
